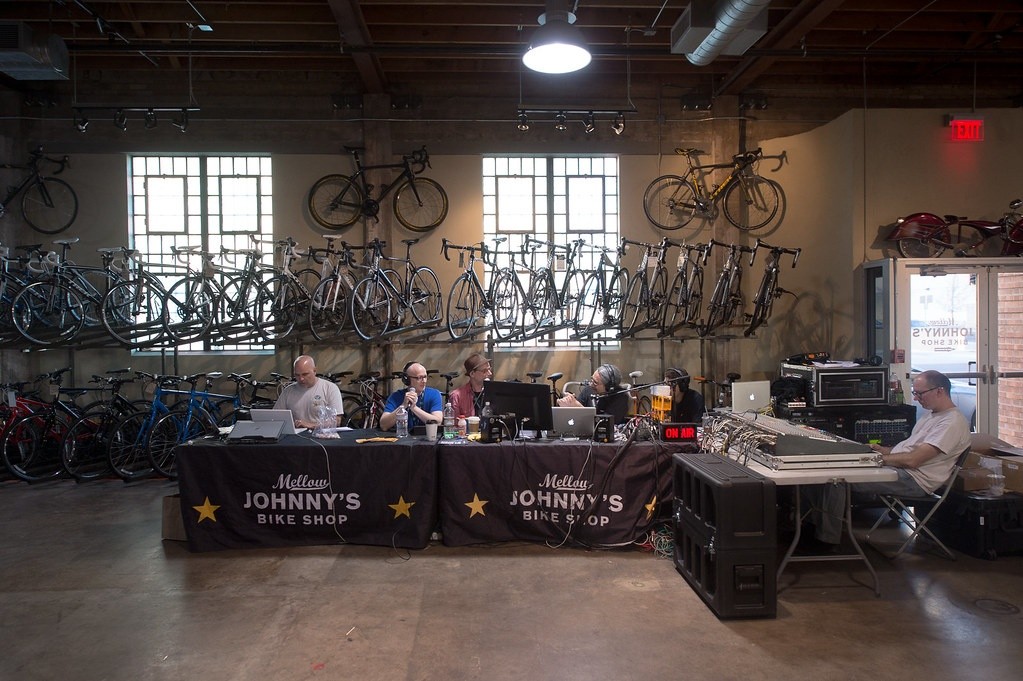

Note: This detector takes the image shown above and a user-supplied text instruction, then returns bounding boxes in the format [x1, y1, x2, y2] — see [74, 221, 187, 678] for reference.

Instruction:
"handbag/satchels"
[772, 377, 806, 402]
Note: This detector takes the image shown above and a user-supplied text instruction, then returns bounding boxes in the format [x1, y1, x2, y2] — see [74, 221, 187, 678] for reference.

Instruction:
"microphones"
[589, 390, 597, 408]
[482, 378, 490, 391]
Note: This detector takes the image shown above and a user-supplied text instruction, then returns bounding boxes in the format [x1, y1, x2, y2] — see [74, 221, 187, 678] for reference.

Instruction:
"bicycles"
[0, 369, 562, 483]
[0, 146, 79, 235]
[693, 372, 742, 422]
[885, 199, 1023, 258]
[307, 146, 449, 232]
[642, 148, 779, 231]
[0, 233, 802, 346]
[628, 371, 651, 419]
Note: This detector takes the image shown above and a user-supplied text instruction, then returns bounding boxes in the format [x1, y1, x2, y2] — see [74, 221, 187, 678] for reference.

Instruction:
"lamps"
[517, 25, 638, 135]
[522, 0, 593, 74]
[72, 20, 200, 133]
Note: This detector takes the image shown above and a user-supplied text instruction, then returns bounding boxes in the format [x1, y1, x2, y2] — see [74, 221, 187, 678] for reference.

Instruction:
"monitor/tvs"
[483, 380, 553, 440]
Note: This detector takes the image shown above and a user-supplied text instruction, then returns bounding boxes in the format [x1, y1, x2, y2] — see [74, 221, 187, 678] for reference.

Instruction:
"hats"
[464, 354, 492, 377]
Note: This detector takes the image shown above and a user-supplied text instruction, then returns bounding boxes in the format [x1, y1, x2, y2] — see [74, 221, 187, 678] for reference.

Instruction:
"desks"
[438, 424, 700, 547]
[706, 437, 899, 600]
[176, 427, 444, 553]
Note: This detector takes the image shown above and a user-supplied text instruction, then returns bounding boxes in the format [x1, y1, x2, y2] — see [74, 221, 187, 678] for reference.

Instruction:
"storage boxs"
[944, 433, 1023, 494]
[780, 363, 890, 406]
[162, 493, 188, 541]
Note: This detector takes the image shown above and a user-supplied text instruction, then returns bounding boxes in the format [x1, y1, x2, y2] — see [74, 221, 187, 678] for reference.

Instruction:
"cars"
[910, 365, 977, 432]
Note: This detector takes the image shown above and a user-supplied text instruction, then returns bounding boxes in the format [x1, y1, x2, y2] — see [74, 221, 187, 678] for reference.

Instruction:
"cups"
[468, 417, 480, 433]
[425, 420, 438, 441]
[986, 474, 1006, 497]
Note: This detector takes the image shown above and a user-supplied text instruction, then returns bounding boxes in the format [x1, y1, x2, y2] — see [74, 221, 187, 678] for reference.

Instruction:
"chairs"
[867, 445, 971, 560]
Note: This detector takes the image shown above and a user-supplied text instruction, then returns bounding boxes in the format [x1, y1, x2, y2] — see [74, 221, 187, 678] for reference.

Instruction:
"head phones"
[401, 361, 418, 386]
[667, 367, 689, 393]
[855, 356, 883, 366]
[604, 364, 618, 393]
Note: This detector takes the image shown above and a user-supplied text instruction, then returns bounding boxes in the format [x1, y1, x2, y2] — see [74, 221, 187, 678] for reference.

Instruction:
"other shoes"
[791, 524, 816, 538]
[796, 537, 833, 556]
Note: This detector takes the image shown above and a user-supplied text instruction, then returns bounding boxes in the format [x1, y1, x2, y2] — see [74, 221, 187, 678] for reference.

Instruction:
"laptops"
[732, 381, 770, 414]
[547, 406, 596, 437]
[250, 409, 308, 435]
[225, 420, 288, 443]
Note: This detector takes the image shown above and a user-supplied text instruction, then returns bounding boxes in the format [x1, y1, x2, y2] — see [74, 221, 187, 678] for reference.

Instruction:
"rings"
[300, 421, 301, 423]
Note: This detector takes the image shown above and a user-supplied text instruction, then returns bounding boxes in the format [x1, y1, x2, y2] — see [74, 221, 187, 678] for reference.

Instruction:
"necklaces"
[473, 395, 484, 408]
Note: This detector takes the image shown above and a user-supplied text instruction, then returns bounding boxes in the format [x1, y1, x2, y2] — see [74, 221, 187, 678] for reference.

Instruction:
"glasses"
[590, 377, 604, 386]
[912, 385, 944, 399]
[475, 367, 493, 374]
[411, 376, 427, 381]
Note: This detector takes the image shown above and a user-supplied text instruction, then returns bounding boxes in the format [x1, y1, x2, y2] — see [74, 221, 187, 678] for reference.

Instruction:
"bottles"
[887, 372, 904, 406]
[458, 415, 467, 438]
[444, 402, 455, 439]
[482, 402, 492, 418]
[395, 406, 408, 438]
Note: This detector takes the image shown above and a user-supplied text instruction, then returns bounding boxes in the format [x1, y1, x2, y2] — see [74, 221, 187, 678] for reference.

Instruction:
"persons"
[663, 368, 704, 423]
[448, 354, 492, 418]
[557, 364, 629, 425]
[813, 370, 970, 555]
[380, 362, 444, 432]
[273, 355, 343, 427]
